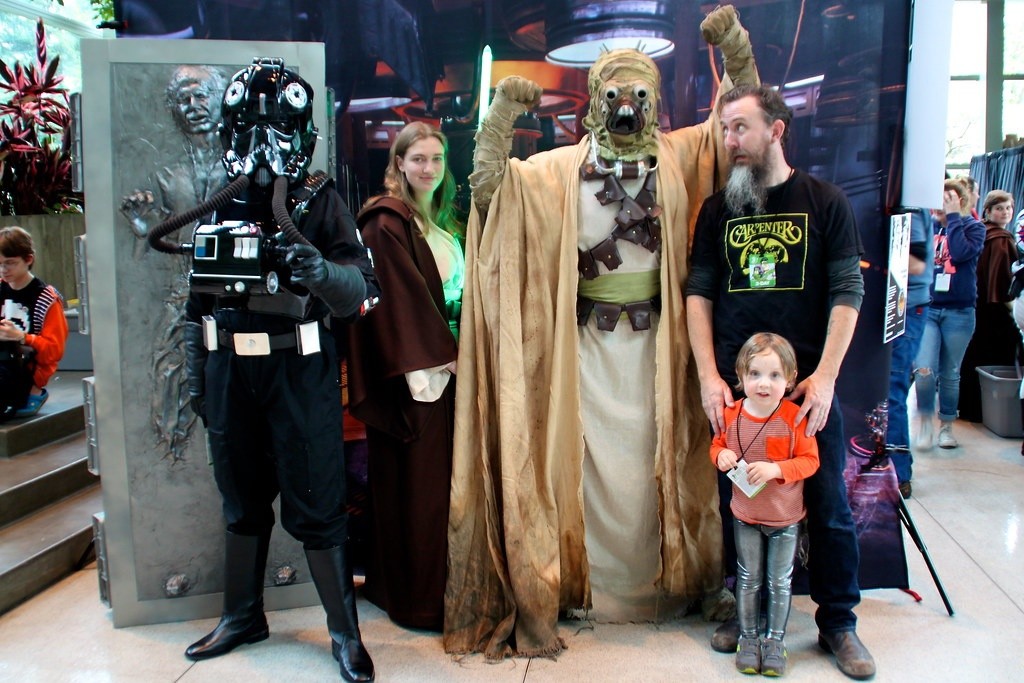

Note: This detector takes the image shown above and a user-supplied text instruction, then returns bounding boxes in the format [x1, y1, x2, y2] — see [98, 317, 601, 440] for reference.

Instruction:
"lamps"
[546, 1, 676, 67]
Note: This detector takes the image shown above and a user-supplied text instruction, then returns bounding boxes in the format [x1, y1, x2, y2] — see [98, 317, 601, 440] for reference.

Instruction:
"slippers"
[14, 389, 48, 418]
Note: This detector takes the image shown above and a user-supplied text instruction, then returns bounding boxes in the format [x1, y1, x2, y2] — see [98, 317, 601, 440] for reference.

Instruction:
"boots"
[305, 543, 374, 683]
[184, 528, 274, 660]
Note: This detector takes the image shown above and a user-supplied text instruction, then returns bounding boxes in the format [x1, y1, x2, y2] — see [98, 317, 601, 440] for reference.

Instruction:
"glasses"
[0, 256, 26, 271]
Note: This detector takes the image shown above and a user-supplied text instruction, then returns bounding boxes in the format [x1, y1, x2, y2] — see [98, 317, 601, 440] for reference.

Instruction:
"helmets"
[220, 58, 318, 191]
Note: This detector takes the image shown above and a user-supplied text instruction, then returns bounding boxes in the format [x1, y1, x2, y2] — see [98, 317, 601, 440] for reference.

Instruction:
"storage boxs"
[976, 363, 1023, 438]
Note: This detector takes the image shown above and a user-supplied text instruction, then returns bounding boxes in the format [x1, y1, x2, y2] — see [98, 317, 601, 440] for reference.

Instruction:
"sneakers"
[735, 633, 762, 675]
[760, 641, 786, 678]
[938, 426, 958, 448]
[915, 418, 933, 451]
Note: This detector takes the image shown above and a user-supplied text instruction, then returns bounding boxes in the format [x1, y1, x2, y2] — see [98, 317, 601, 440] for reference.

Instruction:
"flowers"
[0, 19, 73, 215]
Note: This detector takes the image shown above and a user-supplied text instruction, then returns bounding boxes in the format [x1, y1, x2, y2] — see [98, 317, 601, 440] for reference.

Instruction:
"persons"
[884, 208, 934, 496]
[149, 56, 379, 683]
[117, 67, 234, 469]
[440, 4, 765, 666]
[710, 332, 819, 677]
[0, 225, 70, 417]
[673, 83, 875, 676]
[958, 189, 1024, 421]
[913, 176, 987, 448]
[347, 120, 465, 634]
[967, 178, 981, 220]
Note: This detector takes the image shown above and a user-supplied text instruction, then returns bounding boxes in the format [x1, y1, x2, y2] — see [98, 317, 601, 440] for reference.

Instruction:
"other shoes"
[821, 625, 875, 677]
[898, 480, 913, 500]
[710, 608, 768, 654]
